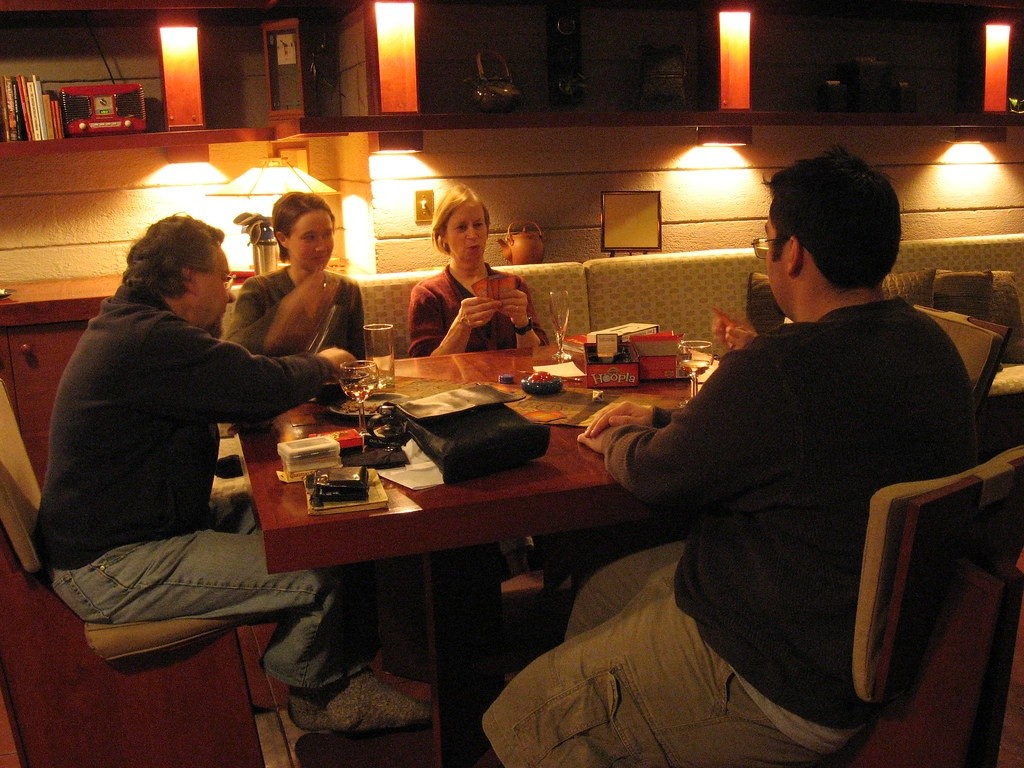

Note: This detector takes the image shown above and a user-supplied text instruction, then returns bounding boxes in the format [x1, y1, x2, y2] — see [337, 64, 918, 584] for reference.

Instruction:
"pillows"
[746, 271, 781, 329]
[876, 272, 934, 306]
[991, 272, 1024, 366]
[941, 270, 989, 317]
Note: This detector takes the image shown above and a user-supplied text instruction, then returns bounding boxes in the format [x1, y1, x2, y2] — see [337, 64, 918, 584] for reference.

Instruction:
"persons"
[34, 217, 431, 731]
[226, 192, 366, 364]
[406, 184, 550, 578]
[713, 315, 794, 351]
[480, 144, 979, 768]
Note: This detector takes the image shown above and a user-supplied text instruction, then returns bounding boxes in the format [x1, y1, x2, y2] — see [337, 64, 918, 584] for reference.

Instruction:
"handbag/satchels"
[367, 385, 550, 485]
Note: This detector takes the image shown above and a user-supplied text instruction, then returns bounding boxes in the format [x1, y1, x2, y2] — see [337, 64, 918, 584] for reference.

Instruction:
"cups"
[363, 323, 396, 389]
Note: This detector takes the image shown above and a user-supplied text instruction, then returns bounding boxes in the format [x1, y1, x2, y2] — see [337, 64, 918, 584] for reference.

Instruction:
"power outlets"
[416, 190, 433, 224]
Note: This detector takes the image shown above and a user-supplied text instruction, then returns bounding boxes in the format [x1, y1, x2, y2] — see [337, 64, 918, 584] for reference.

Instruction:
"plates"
[330, 391, 408, 415]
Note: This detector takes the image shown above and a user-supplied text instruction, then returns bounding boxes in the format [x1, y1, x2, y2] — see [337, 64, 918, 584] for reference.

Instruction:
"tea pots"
[496, 222, 545, 264]
[462, 48, 522, 113]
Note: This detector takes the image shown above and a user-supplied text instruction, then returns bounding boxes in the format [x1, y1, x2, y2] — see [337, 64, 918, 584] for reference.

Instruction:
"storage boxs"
[585, 324, 678, 386]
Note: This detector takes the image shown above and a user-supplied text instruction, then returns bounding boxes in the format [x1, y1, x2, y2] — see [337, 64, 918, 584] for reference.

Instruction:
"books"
[561, 332, 589, 354]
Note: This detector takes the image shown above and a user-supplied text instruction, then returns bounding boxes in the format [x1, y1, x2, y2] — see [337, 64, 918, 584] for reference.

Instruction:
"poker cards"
[494, 383, 513, 394]
[522, 409, 567, 422]
[471, 273, 516, 298]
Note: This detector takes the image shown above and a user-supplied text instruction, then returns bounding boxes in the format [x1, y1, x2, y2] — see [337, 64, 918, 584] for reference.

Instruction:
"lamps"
[697, 126, 754, 146]
[210, 157, 342, 275]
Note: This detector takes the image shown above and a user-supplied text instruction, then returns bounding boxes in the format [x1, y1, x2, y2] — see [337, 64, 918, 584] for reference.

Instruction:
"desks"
[236, 344, 695, 767]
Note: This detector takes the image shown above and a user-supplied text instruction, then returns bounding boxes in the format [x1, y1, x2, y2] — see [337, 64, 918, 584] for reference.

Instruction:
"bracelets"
[514, 317, 533, 335]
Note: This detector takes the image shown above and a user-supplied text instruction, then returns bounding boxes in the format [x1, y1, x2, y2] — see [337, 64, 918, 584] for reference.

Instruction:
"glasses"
[190, 262, 237, 291]
[751, 237, 812, 260]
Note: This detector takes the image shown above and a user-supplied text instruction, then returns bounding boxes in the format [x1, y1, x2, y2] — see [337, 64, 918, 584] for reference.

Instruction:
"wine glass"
[548, 290, 573, 360]
[677, 340, 713, 407]
[340, 360, 379, 437]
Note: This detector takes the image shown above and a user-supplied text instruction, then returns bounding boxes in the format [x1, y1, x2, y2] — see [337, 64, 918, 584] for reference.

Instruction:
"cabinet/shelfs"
[10, 326, 93, 476]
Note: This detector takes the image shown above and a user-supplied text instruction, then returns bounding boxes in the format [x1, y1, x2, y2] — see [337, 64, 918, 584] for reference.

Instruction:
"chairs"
[855, 445, 1023, 767]
[911, 306, 1014, 439]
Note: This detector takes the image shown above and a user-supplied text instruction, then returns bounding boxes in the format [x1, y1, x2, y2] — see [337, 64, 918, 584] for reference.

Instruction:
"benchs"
[218, 261, 586, 356]
[1, 381, 265, 768]
[589, 233, 1024, 394]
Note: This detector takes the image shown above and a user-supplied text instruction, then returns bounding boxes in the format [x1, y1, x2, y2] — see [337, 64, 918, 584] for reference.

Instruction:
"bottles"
[252, 216, 278, 277]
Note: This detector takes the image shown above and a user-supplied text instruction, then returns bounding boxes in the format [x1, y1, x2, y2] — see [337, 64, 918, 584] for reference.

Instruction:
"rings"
[730, 344, 735, 349]
[514, 306, 518, 312]
